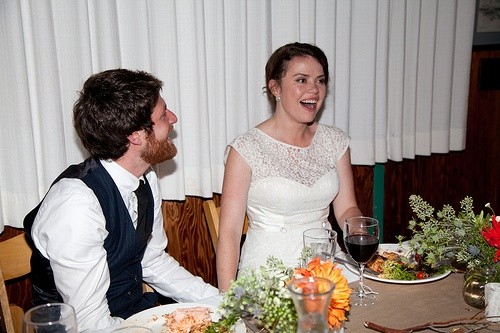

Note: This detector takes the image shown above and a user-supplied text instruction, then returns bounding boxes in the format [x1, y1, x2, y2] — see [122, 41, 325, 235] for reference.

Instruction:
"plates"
[343, 244, 451, 284]
[118, 302, 246, 333]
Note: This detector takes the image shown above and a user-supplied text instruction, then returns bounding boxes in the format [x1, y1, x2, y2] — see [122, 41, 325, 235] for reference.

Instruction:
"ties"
[133, 179, 147, 238]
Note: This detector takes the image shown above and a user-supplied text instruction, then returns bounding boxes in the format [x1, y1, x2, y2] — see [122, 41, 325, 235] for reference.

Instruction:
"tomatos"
[417, 271, 424, 279]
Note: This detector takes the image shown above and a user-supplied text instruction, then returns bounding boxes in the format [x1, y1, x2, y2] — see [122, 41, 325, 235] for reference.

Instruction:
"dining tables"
[92, 215, 500, 333]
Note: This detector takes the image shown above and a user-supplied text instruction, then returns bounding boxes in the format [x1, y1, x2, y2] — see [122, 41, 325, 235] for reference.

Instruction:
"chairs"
[0, 234, 36, 333]
[202, 199, 250, 261]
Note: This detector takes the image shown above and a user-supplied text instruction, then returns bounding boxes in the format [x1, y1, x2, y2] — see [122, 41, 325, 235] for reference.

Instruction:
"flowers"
[203, 260, 351, 333]
[394, 191, 499, 288]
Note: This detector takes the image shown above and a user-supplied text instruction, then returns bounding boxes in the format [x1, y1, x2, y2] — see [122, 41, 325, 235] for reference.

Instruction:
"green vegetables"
[380, 260, 417, 281]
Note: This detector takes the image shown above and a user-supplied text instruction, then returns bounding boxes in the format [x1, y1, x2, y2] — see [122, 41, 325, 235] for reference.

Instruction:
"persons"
[216, 42, 373, 294]
[23, 68, 219, 333]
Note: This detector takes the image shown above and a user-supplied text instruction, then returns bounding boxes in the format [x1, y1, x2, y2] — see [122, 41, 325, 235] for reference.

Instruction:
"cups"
[111, 325, 152, 333]
[24, 302, 79, 333]
[303, 228, 337, 266]
[484, 283, 499, 322]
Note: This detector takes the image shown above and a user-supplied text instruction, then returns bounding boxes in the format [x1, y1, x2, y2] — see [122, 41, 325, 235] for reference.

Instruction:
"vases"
[461, 274, 499, 310]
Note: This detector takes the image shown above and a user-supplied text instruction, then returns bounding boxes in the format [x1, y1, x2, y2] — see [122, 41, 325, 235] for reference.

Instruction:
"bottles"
[288, 277, 336, 333]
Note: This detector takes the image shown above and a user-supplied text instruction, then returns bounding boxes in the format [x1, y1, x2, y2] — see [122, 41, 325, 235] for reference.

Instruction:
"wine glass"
[345, 217, 380, 306]
